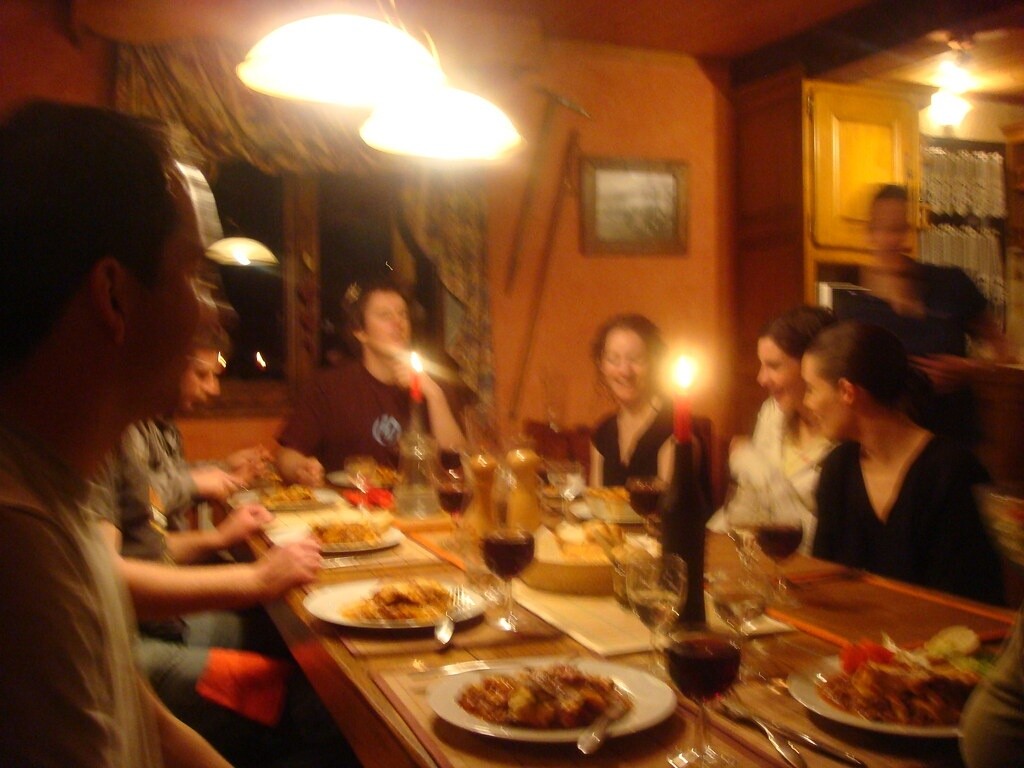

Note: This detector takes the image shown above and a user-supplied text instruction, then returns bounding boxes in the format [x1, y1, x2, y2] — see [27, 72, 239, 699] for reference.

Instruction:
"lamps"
[237, 0, 524, 162]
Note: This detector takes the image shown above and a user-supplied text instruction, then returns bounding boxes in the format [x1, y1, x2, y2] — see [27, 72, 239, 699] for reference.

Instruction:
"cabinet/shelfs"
[738, 58, 941, 514]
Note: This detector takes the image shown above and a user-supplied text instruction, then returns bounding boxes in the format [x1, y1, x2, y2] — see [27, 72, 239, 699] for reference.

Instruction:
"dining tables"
[209, 445, 1024, 768]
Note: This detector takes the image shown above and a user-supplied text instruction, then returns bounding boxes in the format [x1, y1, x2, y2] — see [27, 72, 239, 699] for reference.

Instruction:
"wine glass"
[625, 476, 667, 552]
[626, 555, 691, 681]
[437, 454, 474, 549]
[661, 613, 742, 767]
[756, 504, 806, 608]
[547, 459, 592, 525]
[721, 501, 768, 606]
[481, 517, 533, 631]
[345, 457, 375, 518]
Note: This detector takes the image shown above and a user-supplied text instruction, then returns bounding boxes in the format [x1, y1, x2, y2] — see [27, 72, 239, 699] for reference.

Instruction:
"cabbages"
[879, 623, 992, 679]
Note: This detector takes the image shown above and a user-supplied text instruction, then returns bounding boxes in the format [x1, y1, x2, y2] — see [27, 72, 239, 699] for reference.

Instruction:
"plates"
[574, 500, 641, 527]
[267, 521, 404, 553]
[788, 656, 957, 738]
[423, 654, 678, 745]
[301, 581, 486, 627]
[229, 486, 337, 509]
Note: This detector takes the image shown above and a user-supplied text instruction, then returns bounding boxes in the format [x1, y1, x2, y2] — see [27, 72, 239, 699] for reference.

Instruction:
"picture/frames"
[577, 153, 691, 259]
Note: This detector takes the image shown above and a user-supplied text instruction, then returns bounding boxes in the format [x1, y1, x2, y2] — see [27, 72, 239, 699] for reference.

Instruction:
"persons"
[960, 609, 1024, 768]
[0, 95, 325, 768]
[588, 311, 713, 529]
[701, 184, 1020, 606]
[272, 282, 470, 486]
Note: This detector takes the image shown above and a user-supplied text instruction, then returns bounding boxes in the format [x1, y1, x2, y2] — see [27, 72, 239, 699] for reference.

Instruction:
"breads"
[552, 519, 652, 566]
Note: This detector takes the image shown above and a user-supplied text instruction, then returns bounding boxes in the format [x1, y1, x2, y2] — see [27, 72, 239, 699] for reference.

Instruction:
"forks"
[436, 585, 464, 647]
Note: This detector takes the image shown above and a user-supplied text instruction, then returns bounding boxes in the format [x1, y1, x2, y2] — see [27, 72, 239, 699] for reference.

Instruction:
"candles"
[408, 351, 422, 405]
[668, 355, 700, 443]
